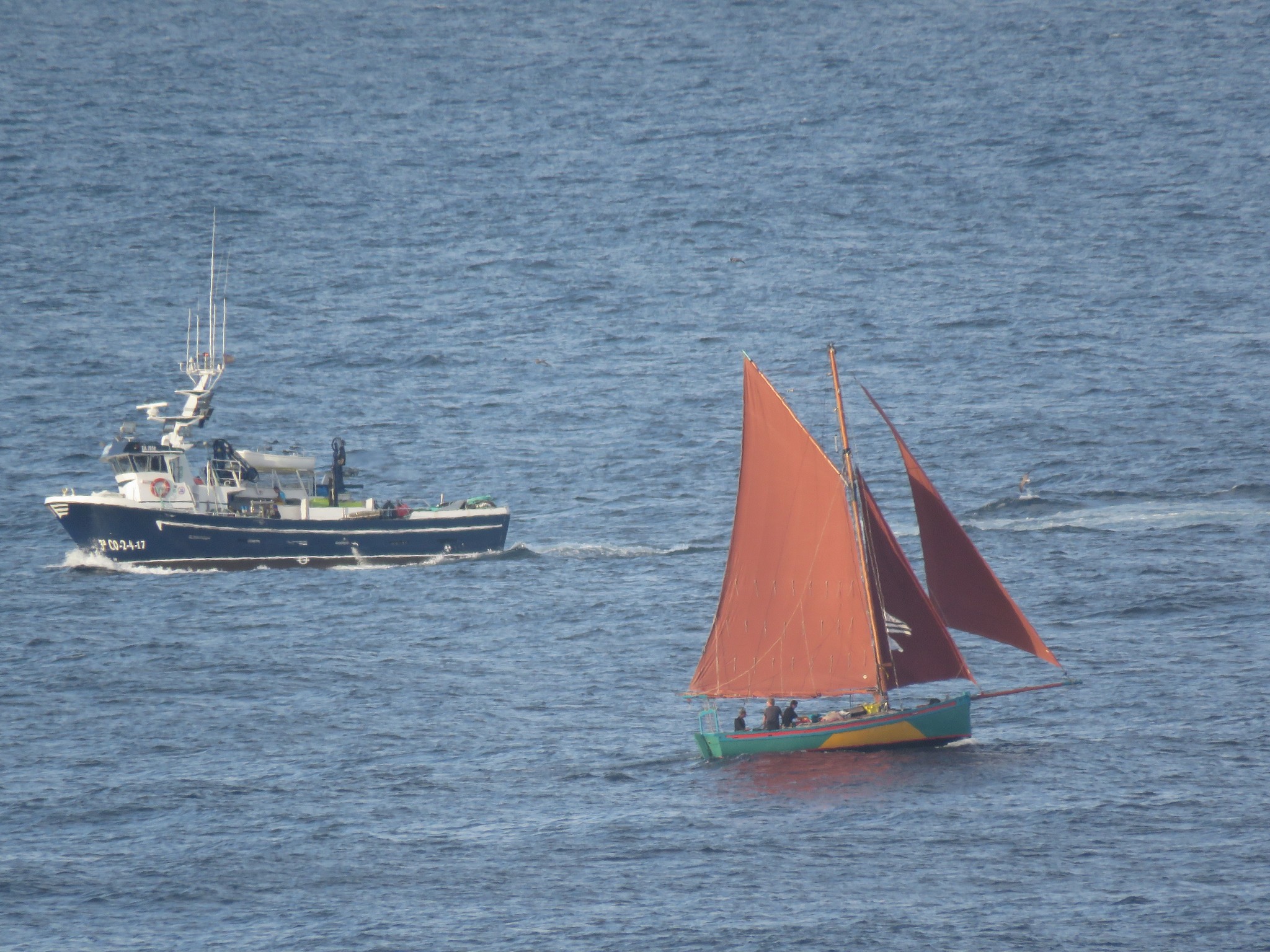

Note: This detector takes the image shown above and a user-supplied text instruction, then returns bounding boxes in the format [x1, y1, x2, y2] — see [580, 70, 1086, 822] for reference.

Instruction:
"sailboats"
[688, 343, 1064, 762]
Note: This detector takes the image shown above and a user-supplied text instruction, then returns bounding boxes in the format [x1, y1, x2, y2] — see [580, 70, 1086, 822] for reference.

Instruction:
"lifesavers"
[151, 476, 170, 498]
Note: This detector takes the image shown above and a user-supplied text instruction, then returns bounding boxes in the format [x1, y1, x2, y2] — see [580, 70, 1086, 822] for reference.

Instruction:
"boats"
[44, 203, 509, 575]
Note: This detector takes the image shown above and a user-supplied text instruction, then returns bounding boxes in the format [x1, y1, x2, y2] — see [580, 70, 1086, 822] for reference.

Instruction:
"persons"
[735, 707, 750, 732]
[272, 485, 285, 518]
[781, 700, 808, 729]
[763, 697, 783, 732]
